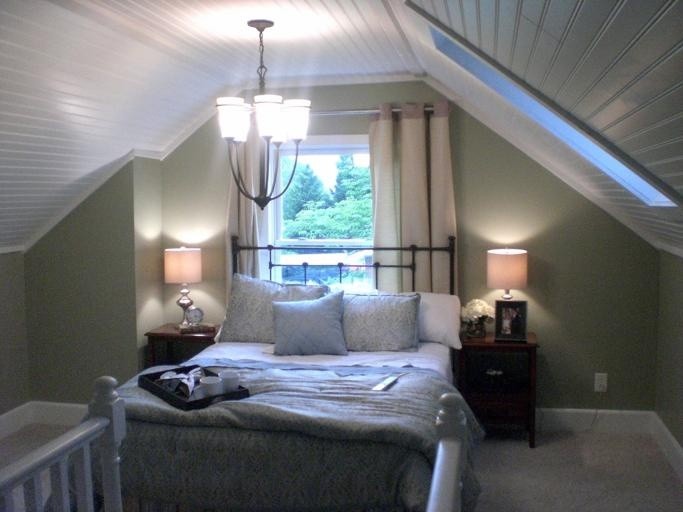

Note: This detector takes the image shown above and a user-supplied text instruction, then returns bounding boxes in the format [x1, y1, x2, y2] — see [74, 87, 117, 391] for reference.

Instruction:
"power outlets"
[594, 373, 608, 393]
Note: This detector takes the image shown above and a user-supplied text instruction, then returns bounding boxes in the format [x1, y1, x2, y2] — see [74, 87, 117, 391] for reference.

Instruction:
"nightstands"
[144, 323, 218, 368]
[450, 331, 540, 448]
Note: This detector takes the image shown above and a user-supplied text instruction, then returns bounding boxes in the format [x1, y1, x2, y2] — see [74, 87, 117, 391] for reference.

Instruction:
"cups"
[200, 376, 223, 397]
[218, 371, 240, 392]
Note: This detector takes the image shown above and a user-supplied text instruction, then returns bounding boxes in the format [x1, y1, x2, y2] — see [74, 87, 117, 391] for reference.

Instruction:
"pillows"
[218, 273, 327, 344]
[341, 294, 421, 352]
[408, 292, 463, 351]
[271, 290, 346, 356]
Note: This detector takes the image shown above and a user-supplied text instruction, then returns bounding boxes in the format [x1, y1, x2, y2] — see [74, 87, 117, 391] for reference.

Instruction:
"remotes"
[372, 376, 398, 391]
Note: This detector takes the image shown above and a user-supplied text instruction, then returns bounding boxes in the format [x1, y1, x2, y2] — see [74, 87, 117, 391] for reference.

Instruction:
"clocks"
[186, 306, 204, 328]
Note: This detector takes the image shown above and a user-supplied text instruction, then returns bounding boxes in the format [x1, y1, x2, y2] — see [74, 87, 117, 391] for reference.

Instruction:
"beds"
[42, 234, 485, 512]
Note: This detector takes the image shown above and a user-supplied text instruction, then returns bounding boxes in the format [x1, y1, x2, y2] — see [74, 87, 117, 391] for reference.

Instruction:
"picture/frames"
[495, 299, 527, 343]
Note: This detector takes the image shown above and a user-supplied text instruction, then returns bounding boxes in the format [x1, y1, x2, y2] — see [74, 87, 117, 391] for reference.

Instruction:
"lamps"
[215, 19, 311, 210]
[165, 245, 204, 334]
[486, 248, 528, 299]
